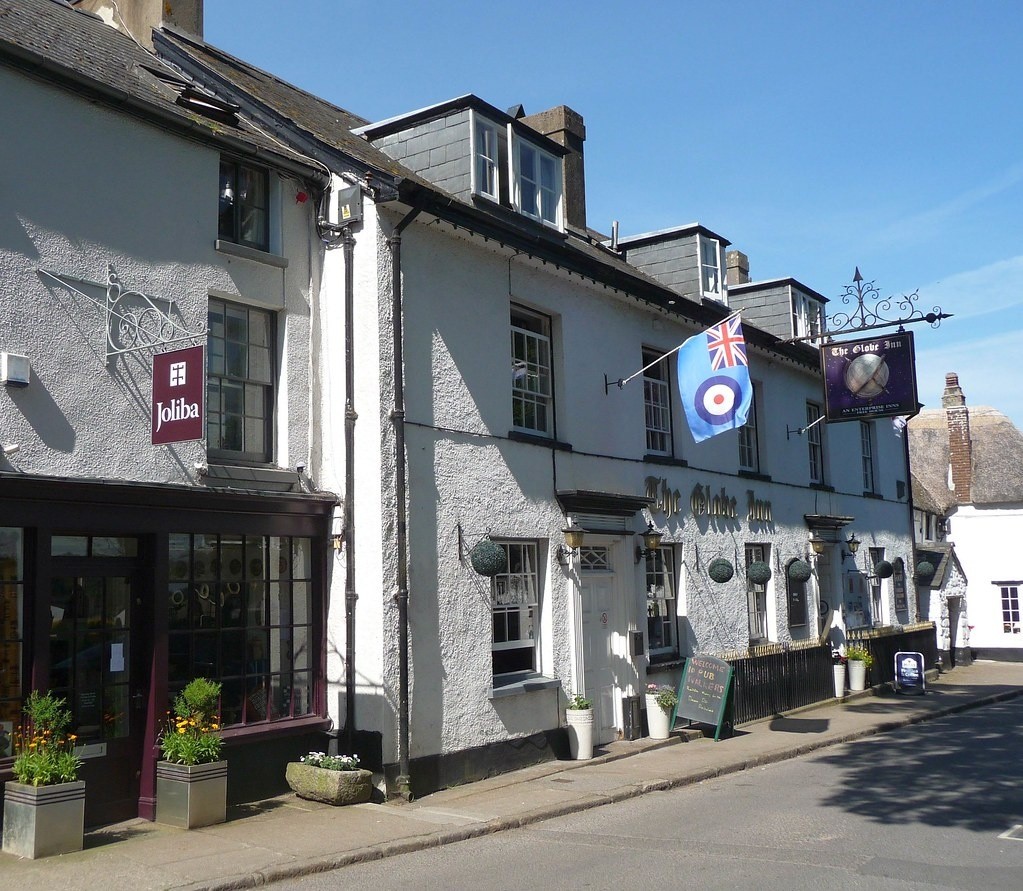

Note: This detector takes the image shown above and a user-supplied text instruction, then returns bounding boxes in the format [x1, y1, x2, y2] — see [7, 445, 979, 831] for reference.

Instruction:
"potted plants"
[566, 687, 595, 761]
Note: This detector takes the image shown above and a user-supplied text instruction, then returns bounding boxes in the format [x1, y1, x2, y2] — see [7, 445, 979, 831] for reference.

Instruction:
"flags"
[677, 314, 753, 443]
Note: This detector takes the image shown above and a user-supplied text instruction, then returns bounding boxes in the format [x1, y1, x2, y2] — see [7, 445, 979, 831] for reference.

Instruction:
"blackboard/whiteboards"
[675, 655, 733, 725]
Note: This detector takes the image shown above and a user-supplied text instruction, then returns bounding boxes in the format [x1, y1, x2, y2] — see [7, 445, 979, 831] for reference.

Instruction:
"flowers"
[831, 643, 873, 672]
[644, 681, 681, 709]
[7, 688, 87, 787]
[300, 751, 361, 771]
[151, 676, 227, 765]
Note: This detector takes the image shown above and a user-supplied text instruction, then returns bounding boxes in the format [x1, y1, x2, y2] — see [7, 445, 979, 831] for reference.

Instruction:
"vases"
[286, 762, 374, 807]
[155, 759, 228, 829]
[832, 659, 866, 698]
[2, 779, 85, 861]
[645, 694, 672, 738]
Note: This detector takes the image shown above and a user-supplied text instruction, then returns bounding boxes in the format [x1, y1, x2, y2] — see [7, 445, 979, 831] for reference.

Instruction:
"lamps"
[841, 532, 861, 560]
[804, 530, 827, 562]
[558, 513, 591, 566]
[634, 520, 664, 564]
